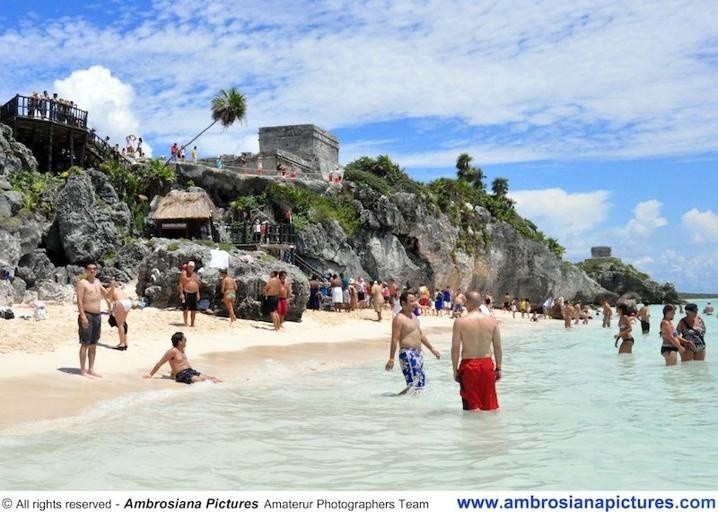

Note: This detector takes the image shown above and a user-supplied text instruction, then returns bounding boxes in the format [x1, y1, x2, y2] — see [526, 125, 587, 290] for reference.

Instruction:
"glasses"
[87, 267, 98, 271]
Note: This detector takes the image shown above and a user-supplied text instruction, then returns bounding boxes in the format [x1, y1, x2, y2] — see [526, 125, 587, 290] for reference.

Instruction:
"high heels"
[111, 344, 128, 351]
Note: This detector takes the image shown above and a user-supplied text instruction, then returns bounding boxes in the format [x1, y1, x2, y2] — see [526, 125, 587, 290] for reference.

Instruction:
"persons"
[150, 332, 222, 383]
[252, 219, 261, 242]
[260, 222, 267, 243]
[278, 272, 290, 324]
[265, 271, 281, 331]
[219, 269, 237, 322]
[105, 280, 132, 351]
[178, 261, 200, 327]
[75, 264, 109, 378]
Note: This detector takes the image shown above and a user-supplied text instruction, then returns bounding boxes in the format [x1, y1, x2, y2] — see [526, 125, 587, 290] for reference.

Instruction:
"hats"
[684, 303, 699, 312]
[187, 261, 195, 267]
